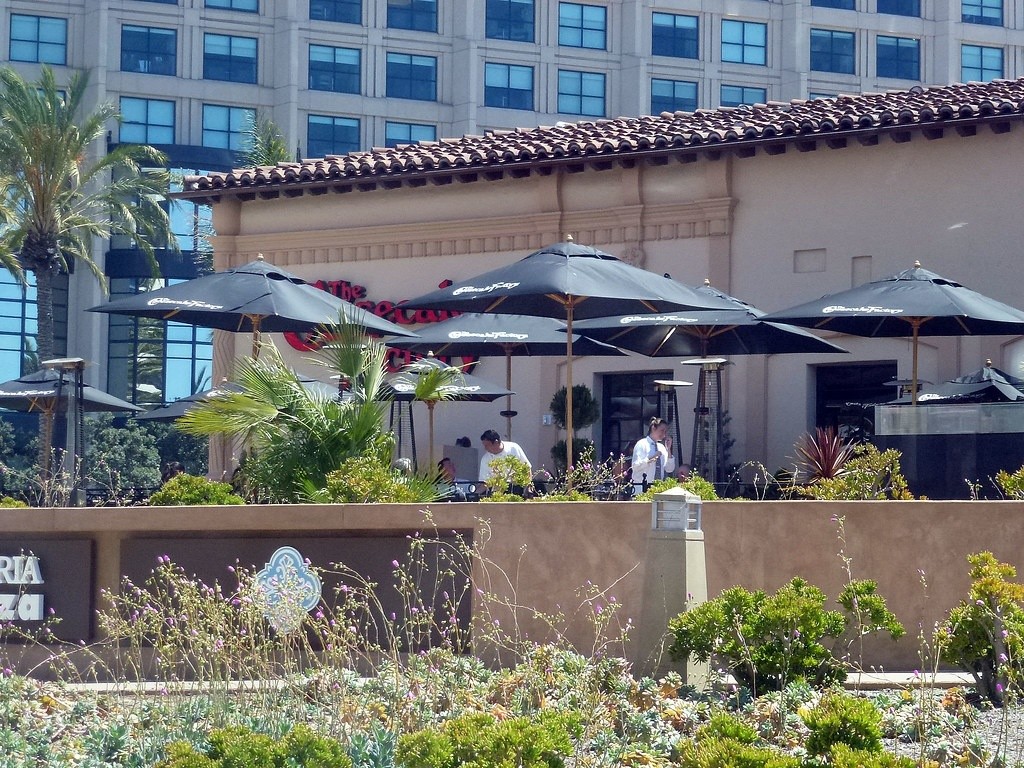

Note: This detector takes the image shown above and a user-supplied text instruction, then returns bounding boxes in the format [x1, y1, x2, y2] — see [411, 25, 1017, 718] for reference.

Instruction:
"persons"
[478, 428, 533, 497]
[231, 465, 251, 500]
[150, 461, 185, 498]
[391, 456, 477, 500]
[631, 416, 676, 501]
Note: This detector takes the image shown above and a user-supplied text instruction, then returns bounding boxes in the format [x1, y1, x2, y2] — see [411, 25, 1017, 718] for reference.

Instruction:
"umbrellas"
[391, 231, 750, 490]
[751, 258, 1024, 407]
[889, 359, 1024, 406]
[0, 366, 149, 486]
[554, 276, 852, 465]
[80, 253, 423, 378]
[353, 349, 518, 481]
[381, 312, 633, 441]
[130, 361, 368, 422]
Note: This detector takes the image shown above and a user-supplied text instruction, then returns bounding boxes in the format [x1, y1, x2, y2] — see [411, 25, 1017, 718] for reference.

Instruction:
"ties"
[652, 443, 661, 480]
